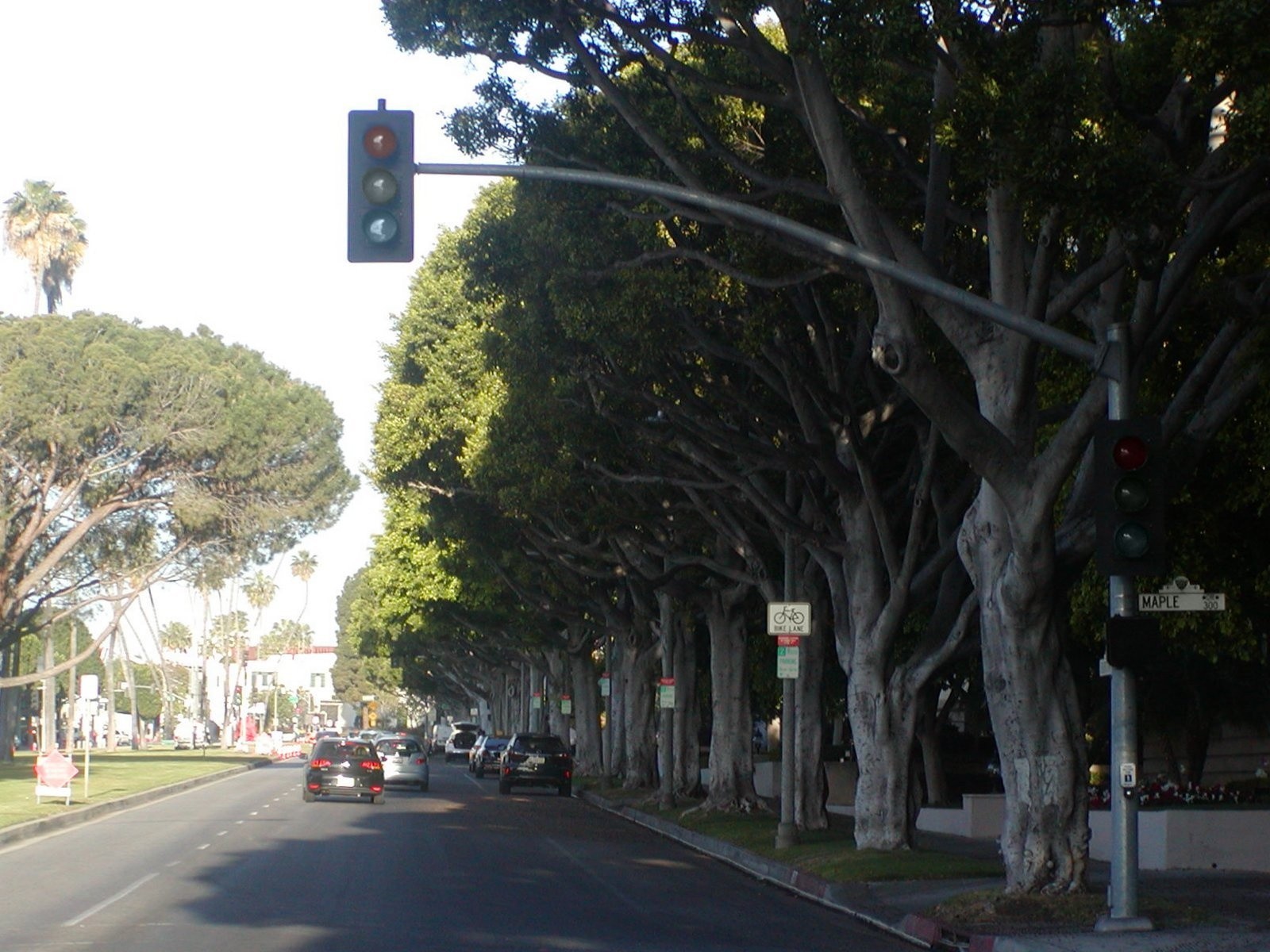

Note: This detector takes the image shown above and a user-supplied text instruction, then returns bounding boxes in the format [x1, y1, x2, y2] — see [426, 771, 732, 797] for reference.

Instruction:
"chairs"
[406, 743, 414, 751]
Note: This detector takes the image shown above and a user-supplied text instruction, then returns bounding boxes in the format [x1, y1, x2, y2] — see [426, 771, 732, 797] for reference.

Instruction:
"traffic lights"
[347, 108, 417, 262]
[1092, 415, 1173, 573]
[1108, 612, 1164, 671]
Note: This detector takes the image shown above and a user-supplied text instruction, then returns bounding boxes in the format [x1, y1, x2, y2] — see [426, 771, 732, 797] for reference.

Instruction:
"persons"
[171, 726, 198, 750]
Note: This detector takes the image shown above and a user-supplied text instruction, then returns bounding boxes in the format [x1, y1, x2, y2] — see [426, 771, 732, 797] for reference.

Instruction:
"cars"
[501, 732, 575, 797]
[314, 722, 510, 791]
[298, 736, 389, 803]
